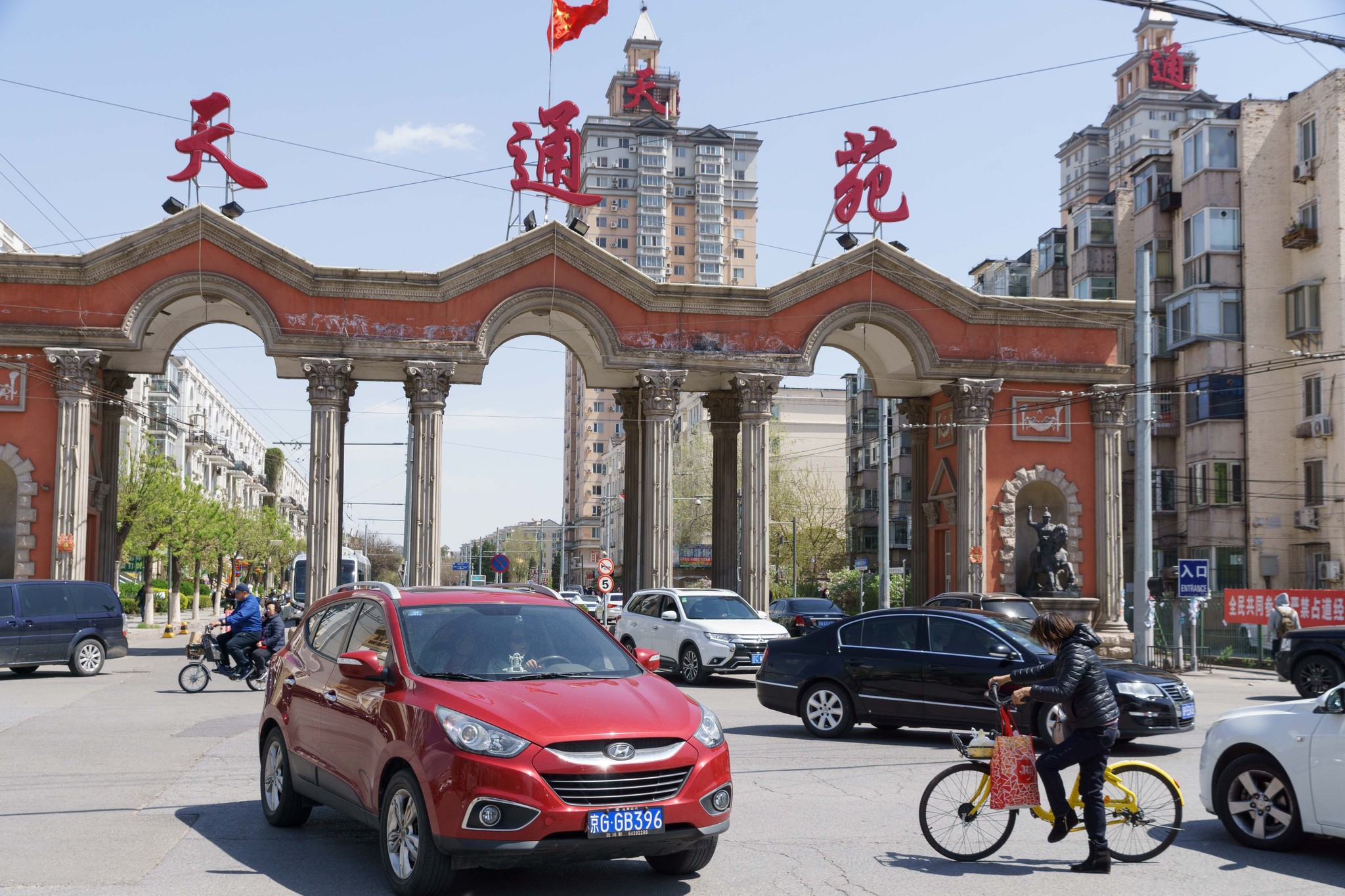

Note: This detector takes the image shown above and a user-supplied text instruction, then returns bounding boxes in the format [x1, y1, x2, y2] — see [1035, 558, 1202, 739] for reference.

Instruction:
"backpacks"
[1274, 606, 1296, 639]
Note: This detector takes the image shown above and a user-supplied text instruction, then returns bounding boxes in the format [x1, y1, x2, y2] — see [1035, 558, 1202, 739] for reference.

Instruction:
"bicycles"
[916, 678, 1186, 862]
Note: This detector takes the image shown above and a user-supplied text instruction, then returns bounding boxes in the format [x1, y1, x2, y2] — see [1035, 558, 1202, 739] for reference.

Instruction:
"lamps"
[221, 200, 246, 219]
[835, 230, 858, 248]
[567, 215, 589, 239]
[889, 240, 911, 253]
[160, 197, 185, 212]
[524, 212, 540, 233]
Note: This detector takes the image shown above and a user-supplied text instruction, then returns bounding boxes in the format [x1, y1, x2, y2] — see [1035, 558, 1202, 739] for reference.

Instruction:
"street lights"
[572, 555, 584, 588]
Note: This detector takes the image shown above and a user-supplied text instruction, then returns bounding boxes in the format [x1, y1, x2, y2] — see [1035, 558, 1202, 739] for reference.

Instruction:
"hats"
[271, 588, 274, 592]
[231, 583, 249, 593]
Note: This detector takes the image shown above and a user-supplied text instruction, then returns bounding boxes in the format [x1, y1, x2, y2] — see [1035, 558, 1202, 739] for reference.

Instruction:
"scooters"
[178, 623, 272, 692]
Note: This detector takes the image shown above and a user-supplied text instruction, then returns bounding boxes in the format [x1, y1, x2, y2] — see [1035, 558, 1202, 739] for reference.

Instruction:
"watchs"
[219, 619, 224, 625]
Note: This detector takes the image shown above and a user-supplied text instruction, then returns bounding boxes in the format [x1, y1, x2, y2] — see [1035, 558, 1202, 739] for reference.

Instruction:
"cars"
[595, 591, 623, 623]
[754, 606, 1196, 748]
[1200, 676, 1344, 848]
[769, 597, 851, 637]
[558, 591, 580, 602]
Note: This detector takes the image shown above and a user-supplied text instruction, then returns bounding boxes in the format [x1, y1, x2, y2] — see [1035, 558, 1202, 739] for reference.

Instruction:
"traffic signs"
[855, 559, 868, 570]
[452, 562, 469, 570]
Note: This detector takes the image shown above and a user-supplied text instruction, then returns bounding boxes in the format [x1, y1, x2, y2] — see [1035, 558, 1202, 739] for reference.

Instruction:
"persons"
[209, 582, 236, 613]
[1027, 506, 1068, 590]
[487, 625, 546, 673]
[252, 601, 286, 680]
[1264, 592, 1302, 681]
[988, 609, 1120, 873]
[134, 584, 146, 625]
[266, 587, 281, 601]
[583, 586, 622, 597]
[209, 583, 262, 683]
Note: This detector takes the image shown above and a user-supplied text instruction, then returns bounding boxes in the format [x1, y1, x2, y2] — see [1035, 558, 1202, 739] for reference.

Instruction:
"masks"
[273, 589, 276, 592]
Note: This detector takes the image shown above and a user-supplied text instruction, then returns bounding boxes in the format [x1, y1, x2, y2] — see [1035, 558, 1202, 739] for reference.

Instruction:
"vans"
[565, 585, 584, 596]
[0, 578, 129, 676]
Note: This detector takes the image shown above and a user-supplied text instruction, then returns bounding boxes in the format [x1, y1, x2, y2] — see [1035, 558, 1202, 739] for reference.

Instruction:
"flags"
[547, 0, 609, 55]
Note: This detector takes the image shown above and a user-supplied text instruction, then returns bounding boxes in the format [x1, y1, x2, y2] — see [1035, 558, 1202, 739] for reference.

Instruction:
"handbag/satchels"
[990, 703, 1042, 810]
[203, 625, 224, 663]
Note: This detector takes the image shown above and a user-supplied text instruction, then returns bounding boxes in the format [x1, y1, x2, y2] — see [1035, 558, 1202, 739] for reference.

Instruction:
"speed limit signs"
[597, 575, 613, 593]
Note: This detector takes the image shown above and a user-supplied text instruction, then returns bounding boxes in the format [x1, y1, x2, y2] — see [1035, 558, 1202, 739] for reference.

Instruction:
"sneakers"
[238, 666, 255, 680]
[212, 664, 232, 674]
[256, 671, 268, 680]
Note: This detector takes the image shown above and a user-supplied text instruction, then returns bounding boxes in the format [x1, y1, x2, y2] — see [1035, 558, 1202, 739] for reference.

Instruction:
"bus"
[289, 545, 372, 624]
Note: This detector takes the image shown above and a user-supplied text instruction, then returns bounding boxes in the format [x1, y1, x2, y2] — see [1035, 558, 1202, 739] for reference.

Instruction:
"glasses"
[266, 608, 275, 611]
[510, 636, 532, 644]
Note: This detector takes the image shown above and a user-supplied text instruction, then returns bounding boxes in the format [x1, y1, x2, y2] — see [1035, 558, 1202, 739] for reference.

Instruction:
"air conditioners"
[1317, 561, 1342, 581]
[1311, 417, 1333, 438]
[1294, 509, 1315, 528]
[1291, 160, 1312, 182]
[571, 142, 737, 555]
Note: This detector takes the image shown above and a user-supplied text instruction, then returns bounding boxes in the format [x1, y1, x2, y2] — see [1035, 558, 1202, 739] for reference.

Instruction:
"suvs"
[1275, 625, 1345, 697]
[256, 581, 734, 896]
[921, 591, 1042, 623]
[614, 586, 792, 685]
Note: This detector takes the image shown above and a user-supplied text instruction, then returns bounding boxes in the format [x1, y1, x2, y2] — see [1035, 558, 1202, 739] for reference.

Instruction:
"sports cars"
[568, 594, 602, 617]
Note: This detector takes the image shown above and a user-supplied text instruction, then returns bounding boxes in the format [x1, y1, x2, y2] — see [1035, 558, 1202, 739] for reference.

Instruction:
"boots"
[1049, 811, 1079, 843]
[1067, 842, 1112, 873]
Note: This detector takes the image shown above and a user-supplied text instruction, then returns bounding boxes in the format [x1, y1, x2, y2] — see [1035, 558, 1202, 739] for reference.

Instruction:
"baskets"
[185, 641, 203, 660]
